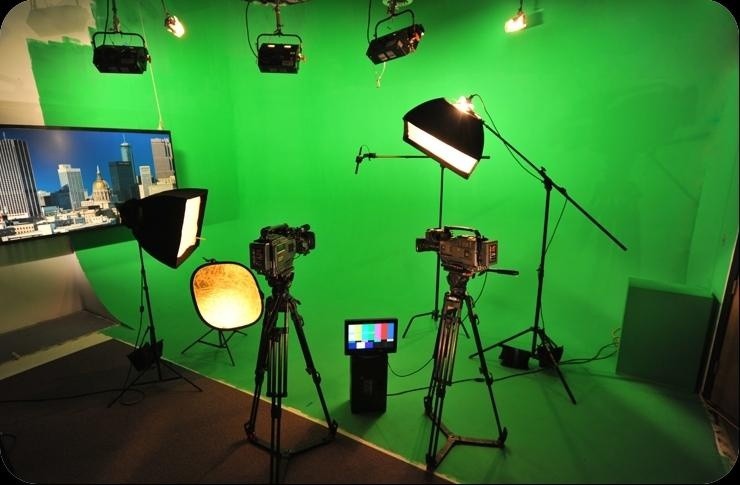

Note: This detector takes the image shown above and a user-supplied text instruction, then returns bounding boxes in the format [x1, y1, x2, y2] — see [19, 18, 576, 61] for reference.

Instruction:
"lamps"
[366, 0, 425, 65]
[245, 0, 302, 73]
[91, 0, 151, 74]
[106, 188, 208, 408]
[402, 94, 628, 404]
[161, 0, 184, 38]
[504, 0, 527, 32]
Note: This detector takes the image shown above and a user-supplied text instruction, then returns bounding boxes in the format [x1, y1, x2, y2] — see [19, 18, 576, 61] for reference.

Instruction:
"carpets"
[0, 336, 458, 485]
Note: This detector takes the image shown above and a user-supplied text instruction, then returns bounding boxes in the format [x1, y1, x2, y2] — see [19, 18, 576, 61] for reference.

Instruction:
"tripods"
[182, 327, 247, 367]
[424, 290, 505, 475]
[469, 180, 575, 402]
[245, 300, 336, 485]
[107, 245, 202, 408]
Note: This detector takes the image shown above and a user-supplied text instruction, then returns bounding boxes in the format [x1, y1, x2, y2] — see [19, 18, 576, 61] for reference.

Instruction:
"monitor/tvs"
[0, 124, 179, 246]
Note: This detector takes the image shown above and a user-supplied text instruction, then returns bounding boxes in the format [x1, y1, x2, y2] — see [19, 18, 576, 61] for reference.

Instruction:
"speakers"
[616, 277, 714, 388]
